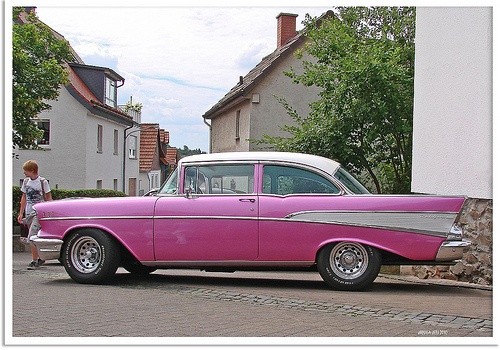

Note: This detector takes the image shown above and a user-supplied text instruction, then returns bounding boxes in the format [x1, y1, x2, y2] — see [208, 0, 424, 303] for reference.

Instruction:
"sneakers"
[27, 261, 40, 269]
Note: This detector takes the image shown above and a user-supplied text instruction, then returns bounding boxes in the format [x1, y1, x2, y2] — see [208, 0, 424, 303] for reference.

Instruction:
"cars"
[29, 151, 474, 292]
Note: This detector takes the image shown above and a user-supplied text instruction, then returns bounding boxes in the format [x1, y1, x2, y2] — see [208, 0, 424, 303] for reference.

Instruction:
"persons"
[18, 160, 52, 270]
